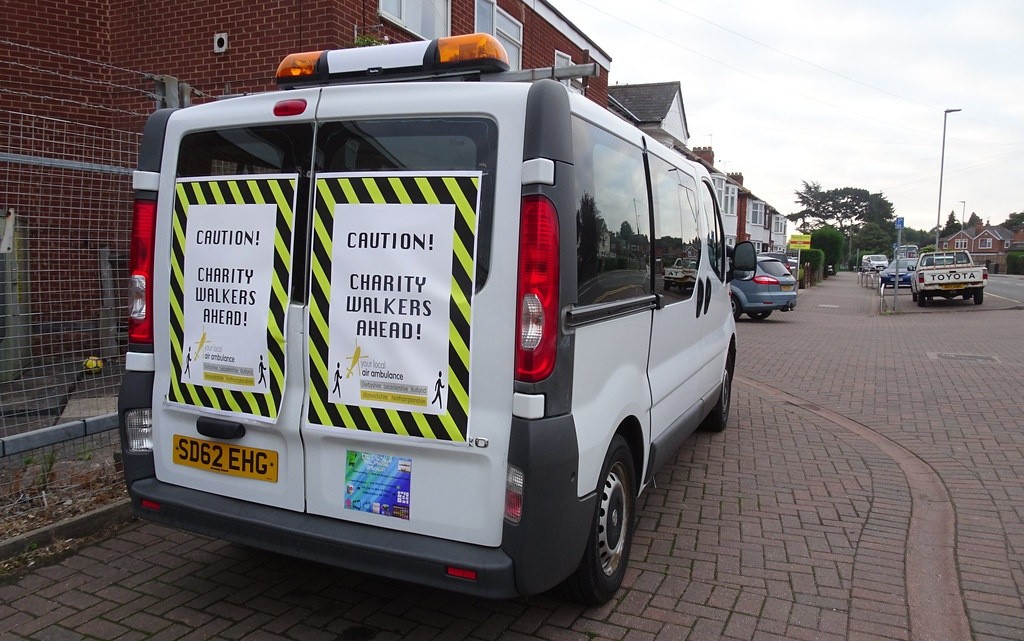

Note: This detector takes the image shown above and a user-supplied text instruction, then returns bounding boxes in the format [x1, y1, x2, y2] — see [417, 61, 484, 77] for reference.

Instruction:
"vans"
[862, 254, 890, 273]
[119, 33, 757, 610]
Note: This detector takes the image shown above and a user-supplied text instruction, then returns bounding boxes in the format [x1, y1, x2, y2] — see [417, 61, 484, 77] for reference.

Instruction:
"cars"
[879, 257, 921, 288]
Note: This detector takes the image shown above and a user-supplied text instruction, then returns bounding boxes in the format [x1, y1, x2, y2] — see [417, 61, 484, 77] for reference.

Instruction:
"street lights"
[934, 109, 962, 250]
[959, 200, 965, 246]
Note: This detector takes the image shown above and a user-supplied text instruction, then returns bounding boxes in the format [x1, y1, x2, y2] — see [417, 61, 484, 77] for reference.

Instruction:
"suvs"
[756, 251, 791, 275]
[729, 257, 799, 321]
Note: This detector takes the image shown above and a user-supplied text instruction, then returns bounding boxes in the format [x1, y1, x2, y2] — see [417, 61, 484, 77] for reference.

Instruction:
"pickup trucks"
[661, 255, 698, 292]
[907, 249, 990, 308]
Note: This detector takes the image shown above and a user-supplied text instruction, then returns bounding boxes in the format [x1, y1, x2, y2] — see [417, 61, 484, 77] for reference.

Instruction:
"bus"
[893, 244, 920, 259]
